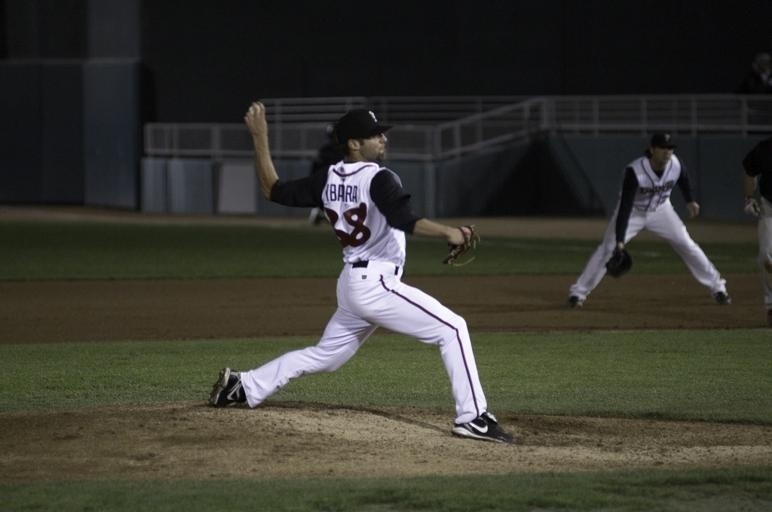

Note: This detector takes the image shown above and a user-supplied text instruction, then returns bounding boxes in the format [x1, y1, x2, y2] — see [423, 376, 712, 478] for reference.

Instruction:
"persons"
[743, 134, 771, 317]
[563, 133, 732, 309]
[209, 95, 514, 446]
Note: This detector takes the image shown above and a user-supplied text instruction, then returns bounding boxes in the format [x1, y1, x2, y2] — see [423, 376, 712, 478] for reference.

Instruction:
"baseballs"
[249, 104, 261, 117]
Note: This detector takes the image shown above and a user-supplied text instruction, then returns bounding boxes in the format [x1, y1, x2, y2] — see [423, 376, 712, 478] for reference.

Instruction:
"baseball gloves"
[443, 224, 481, 266]
[605, 248, 632, 278]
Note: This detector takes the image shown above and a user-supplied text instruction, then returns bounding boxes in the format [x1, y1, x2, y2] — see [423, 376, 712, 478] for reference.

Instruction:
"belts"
[352, 260, 399, 275]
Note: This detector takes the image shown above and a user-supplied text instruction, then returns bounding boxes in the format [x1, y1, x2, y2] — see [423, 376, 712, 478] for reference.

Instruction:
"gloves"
[743, 196, 761, 218]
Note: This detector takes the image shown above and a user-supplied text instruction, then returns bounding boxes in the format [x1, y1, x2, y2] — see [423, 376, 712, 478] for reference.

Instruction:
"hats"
[337, 109, 392, 144]
[651, 133, 677, 149]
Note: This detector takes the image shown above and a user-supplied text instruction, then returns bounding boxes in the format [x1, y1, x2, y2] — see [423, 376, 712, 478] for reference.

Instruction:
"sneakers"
[207, 366, 248, 409]
[451, 411, 511, 443]
[570, 296, 584, 308]
[712, 291, 732, 305]
